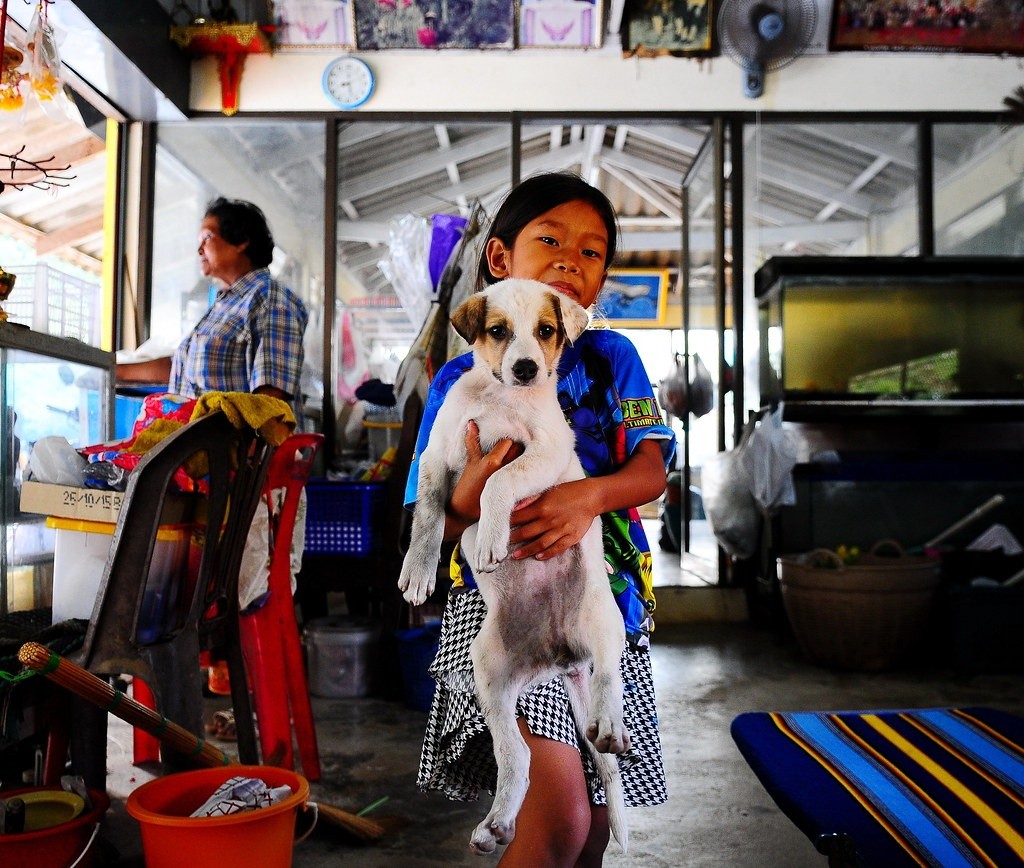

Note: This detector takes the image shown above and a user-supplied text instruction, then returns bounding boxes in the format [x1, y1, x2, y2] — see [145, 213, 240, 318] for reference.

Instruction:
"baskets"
[775, 538, 942, 672]
[304, 477, 384, 555]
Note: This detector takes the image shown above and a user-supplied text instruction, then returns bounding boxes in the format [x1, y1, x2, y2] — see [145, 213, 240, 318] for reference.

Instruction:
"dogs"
[397, 276, 635, 860]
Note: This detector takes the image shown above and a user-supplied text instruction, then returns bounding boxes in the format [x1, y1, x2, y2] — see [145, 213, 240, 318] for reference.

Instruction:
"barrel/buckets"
[302, 613, 372, 702]
[395, 618, 442, 713]
[124, 765, 319, 868]
[0, 785, 112, 868]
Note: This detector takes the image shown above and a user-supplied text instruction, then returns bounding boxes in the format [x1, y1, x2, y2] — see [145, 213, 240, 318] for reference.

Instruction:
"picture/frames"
[587, 269, 671, 326]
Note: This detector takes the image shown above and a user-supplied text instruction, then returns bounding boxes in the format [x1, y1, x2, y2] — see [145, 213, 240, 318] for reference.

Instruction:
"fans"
[716, 0, 820, 98]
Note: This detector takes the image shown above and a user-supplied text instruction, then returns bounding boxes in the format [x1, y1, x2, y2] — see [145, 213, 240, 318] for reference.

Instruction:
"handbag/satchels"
[658, 353, 685, 421]
[700, 402, 798, 555]
[690, 353, 713, 418]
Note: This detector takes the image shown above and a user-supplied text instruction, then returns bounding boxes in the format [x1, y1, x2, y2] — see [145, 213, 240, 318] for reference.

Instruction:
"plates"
[2, 790, 84, 831]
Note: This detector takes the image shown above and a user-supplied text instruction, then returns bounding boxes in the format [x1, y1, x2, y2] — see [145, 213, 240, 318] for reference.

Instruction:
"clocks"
[322, 56, 373, 108]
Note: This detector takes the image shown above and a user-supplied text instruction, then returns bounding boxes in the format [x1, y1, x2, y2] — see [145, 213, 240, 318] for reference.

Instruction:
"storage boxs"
[361, 420, 403, 461]
[45, 514, 191, 646]
[754, 252, 1023, 400]
[20, 483, 206, 527]
[303, 481, 388, 554]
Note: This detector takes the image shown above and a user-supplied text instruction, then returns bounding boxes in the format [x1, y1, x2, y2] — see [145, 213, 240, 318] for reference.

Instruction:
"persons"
[404, 173, 676, 868]
[169, 196, 310, 433]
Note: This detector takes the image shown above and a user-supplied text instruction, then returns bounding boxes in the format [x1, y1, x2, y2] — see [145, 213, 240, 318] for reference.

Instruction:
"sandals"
[204, 708, 259, 741]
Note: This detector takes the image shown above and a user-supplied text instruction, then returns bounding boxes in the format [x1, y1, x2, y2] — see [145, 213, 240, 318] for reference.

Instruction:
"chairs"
[293, 391, 423, 662]
[0, 409, 273, 784]
[132, 433, 326, 783]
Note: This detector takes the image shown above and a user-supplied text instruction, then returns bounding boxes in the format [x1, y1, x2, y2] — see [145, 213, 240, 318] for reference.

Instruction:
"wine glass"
[0, 273, 16, 323]
